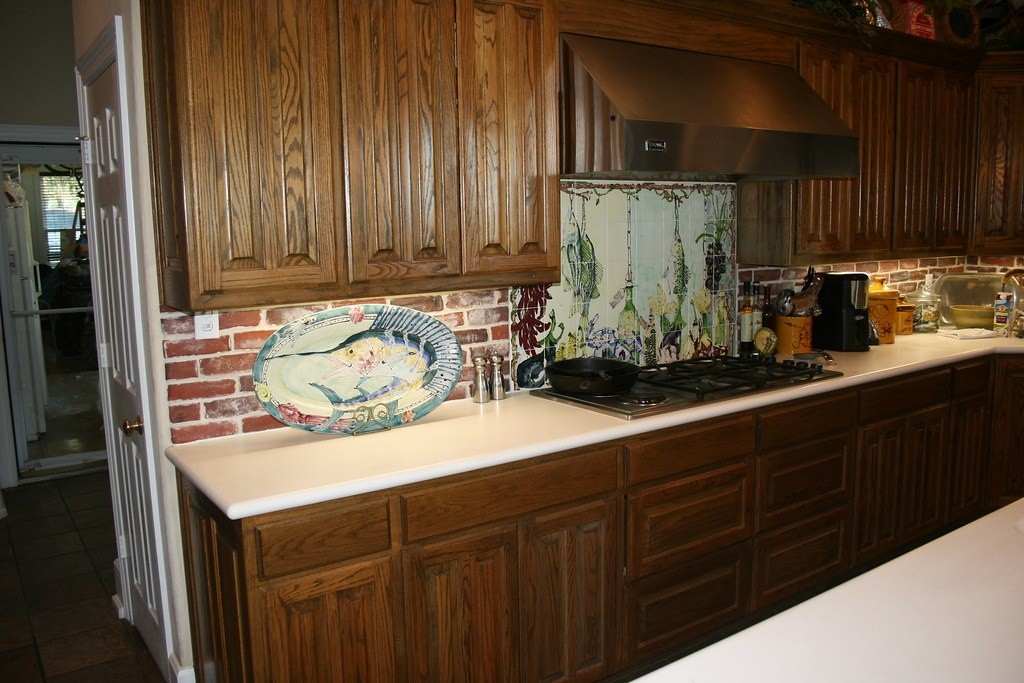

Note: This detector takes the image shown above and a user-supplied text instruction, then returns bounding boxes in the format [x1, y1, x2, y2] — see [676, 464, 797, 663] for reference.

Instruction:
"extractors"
[559, 32, 861, 182]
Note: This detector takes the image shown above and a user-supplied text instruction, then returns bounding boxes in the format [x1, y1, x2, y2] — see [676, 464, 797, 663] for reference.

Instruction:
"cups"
[776, 315, 812, 355]
[867, 277, 899, 344]
[896, 294, 917, 335]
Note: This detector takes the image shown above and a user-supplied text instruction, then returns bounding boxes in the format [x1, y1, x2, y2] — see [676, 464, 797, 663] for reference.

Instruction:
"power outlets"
[193, 314, 220, 340]
[926, 274, 933, 289]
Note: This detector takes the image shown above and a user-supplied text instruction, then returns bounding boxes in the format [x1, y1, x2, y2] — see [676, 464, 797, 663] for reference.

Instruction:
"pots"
[544, 358, 641, 395]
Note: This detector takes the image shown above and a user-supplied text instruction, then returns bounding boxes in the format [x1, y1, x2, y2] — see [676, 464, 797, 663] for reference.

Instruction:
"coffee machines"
[812, 270, 872, 352]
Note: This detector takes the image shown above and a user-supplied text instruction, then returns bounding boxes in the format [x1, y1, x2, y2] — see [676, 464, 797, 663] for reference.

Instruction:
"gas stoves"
[529, 355, 844, 419]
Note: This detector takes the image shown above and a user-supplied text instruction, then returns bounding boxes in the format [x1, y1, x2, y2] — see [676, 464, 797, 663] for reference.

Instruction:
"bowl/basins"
[951, 305, 994, 330]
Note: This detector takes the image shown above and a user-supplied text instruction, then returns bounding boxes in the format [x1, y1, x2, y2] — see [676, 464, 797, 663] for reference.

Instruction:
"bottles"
[487, 354, 506, 399]
[906, 284, 941, 333]
[751, 281, 762, 353]
[762, 286, 776, 333]
[741, 281, 751, 353]
[472, 356, 491, 403]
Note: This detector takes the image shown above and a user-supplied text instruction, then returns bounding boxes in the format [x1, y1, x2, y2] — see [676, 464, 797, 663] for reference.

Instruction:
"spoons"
[776, 276, 823, 317]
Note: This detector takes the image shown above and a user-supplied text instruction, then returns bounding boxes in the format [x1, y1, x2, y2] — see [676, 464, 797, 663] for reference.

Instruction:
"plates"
[254, 304, 464, 435]
[754, 328, 777, 355]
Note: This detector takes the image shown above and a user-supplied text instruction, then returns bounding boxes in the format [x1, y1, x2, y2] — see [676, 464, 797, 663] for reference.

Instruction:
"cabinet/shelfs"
[967, 69, 1024, 257]
[141, 0, 562, 312]
[749, 385, 861, 615]
[176, 438, 624, 683]
[945, 357, 996, 526]
[984, 355, 1024, 508]
[848, 364, 953, 570]
[736, 34, 897, 268]
[625, 410, 757, 669]
[891, 58, 977, 260]
